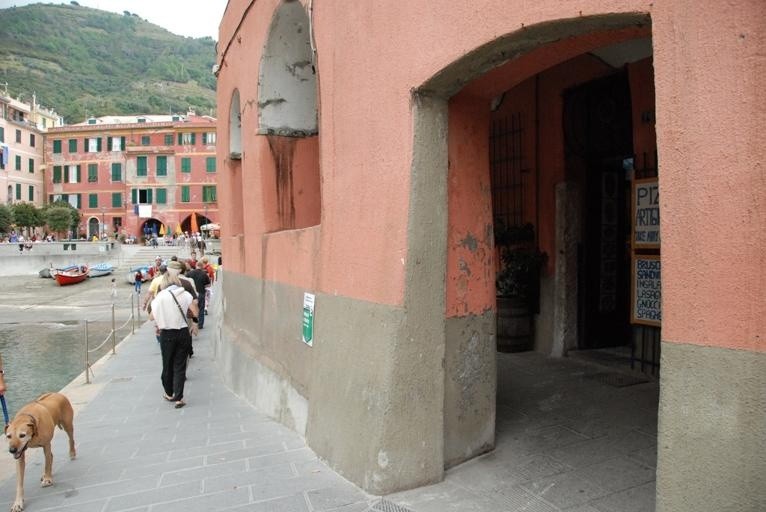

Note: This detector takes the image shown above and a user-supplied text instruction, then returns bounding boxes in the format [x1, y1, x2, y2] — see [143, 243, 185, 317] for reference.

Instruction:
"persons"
[145, 231, 217, 257]
[142, 252, 215, 408]
[110, 279, 117, 298]
[80, 232, 98, 241]
[135, 270, 143, 296]
[2, 229, 56, 250]
[66, 231, 72, 251]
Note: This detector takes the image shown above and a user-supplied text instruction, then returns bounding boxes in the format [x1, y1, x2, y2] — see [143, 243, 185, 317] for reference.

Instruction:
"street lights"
[101, 204, 107, 241]
[203, 205, 211, 240]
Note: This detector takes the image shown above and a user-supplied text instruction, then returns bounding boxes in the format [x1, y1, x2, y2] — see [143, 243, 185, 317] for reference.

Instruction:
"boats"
[38, 263, 112, 286]
[127, 263, 160, 284]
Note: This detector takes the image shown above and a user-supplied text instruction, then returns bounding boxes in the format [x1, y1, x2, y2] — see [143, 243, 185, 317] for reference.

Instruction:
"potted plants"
[494, 221, 549, 352]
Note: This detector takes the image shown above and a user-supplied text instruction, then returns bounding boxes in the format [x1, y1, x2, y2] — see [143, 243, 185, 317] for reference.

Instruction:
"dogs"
[4, 392, 77, 512]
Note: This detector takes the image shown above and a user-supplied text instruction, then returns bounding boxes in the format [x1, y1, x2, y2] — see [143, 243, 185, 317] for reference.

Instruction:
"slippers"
[175, 401, 185, 408]
[163, 392, 174, 401]
